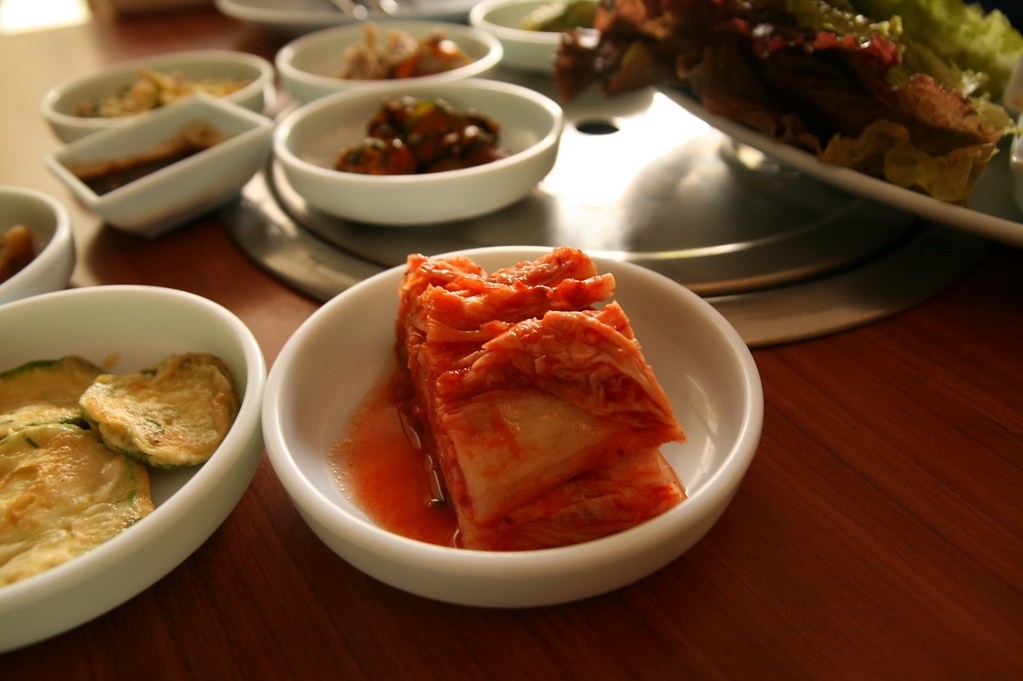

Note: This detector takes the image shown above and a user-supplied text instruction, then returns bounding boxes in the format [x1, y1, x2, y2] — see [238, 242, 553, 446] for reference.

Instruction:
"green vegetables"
[682, 0, 1023, 205]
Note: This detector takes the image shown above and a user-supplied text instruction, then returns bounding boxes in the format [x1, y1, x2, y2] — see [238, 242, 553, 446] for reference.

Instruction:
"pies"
[0, 351, 240, 587]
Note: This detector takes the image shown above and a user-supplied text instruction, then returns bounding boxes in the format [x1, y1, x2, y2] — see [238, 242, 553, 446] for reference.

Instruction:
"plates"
[653, 83, 1022, 246]
[213, 0, 479, 40]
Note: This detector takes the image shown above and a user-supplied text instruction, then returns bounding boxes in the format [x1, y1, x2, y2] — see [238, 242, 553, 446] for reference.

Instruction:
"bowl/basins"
[273, 79, 564, 226]
[0, 186, 75, 304]
[42, 50, 273, 144]
[261, 245, 764, 611]
[1, 286, 267, 651]
[469, 2, 603, 76]
[35, 91, 275, 239]
[274, 21, 503, 107]
